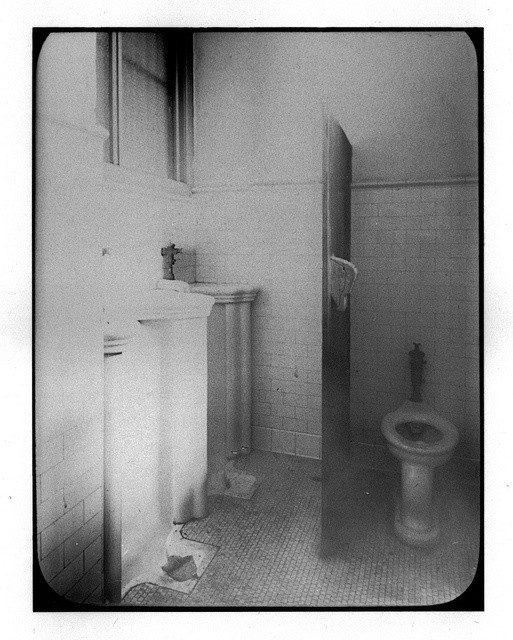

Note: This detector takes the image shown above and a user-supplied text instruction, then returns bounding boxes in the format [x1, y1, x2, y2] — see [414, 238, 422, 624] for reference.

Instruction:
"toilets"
[381, 340, 458, 543]
